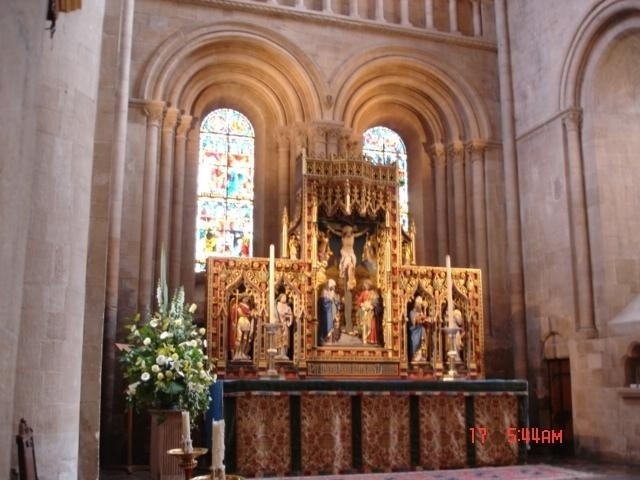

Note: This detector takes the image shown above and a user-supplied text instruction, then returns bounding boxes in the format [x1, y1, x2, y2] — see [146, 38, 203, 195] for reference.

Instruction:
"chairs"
[14, 416, 41, 480]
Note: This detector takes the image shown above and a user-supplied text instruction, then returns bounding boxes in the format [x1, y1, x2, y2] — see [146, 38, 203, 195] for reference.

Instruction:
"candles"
[180, 410, 227, 469]
[267, 205, 455, 327]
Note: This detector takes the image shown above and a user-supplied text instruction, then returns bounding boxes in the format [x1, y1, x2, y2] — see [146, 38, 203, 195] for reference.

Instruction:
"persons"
[231, 292, 256, 361]
[263, 293, 294, 362]
[317, 280, 344, 345]
[445, 305, 466, 360]
[356, 280, 380, 345]
[409, 297, 429, 359]
[328, 225, 370, 289]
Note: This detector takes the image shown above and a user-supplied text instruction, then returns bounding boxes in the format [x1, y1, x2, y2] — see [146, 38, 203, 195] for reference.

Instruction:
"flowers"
[109, 279, 219, 433]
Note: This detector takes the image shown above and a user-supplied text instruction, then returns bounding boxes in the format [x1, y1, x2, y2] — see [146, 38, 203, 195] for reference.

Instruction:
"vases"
[151, 405, 188, 480]
